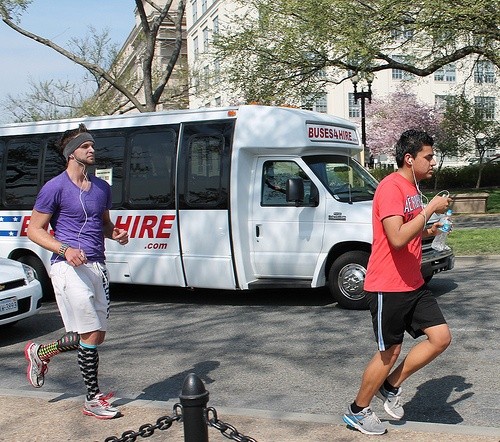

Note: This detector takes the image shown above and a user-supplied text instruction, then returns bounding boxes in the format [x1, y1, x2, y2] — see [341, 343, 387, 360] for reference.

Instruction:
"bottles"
[431, 210, 453, 252]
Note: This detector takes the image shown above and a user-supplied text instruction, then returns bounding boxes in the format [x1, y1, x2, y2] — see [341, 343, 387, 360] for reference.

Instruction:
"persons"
[25, 123, 129, 420]
[342, 129, 453, 436]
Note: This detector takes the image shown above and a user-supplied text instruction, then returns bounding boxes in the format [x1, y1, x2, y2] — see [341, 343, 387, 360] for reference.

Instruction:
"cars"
[0, 256, 45, 329]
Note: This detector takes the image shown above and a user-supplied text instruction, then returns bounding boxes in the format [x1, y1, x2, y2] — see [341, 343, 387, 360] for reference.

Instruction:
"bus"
[0, 104, 456, 310]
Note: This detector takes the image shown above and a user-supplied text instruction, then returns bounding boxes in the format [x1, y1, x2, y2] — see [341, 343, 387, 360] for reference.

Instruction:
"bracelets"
[58, 244, 69, 259]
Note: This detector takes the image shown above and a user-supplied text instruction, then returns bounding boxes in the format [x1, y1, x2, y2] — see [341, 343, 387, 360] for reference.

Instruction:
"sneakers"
[25, 341, 51, 388]
[82, 392, 121, 419]
[342, 405, 388, 435]
[375, 384, 404, 419]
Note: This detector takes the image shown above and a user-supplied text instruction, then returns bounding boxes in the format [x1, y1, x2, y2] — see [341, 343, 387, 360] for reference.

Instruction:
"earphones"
[409, 158, 412, 163]
[71, 154, 74, 158]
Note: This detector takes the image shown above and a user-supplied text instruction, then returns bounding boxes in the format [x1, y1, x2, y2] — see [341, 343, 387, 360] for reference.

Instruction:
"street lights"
[350, 72, 375, 147]
